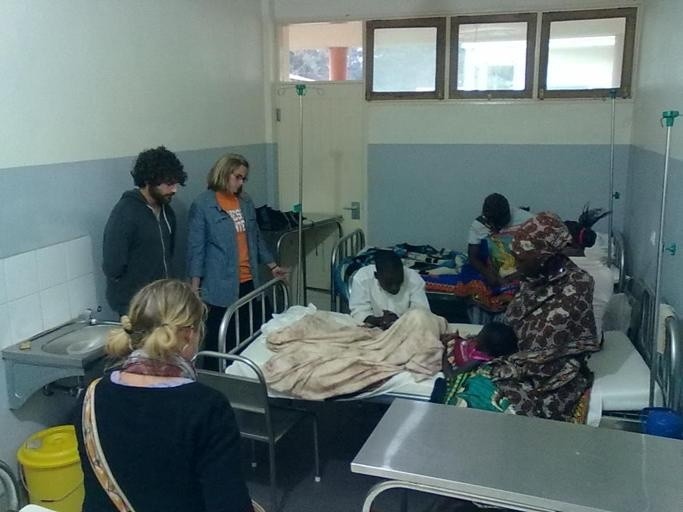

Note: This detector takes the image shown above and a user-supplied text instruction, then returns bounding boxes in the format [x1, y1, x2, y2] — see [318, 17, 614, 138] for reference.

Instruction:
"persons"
[103, 143, 189, 316]
[348, 249, 432, 332]
[439, 210, 599, 423]
[459, 193, 520, 326]
[184, 147, 295, 376]
[73, 277, 260, 511]
[438, 319, 519, 381]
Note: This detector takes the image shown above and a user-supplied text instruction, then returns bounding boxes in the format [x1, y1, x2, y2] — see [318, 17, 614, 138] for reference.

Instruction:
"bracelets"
[270, 263, 278, 270]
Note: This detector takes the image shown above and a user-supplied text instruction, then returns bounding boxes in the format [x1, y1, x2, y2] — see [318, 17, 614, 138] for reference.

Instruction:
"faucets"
[85, 307, 97, 325]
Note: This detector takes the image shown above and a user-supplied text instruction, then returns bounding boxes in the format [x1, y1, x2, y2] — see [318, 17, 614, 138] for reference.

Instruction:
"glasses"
[231, 171, 248, 183]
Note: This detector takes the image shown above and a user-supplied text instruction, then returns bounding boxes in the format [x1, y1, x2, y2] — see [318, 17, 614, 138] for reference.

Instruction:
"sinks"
[41, 325, 122, 355]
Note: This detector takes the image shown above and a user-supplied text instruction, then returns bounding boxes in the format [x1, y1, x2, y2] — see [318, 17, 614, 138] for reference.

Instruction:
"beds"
[327, 227, 627, 320]
[214, 276, 682, 437]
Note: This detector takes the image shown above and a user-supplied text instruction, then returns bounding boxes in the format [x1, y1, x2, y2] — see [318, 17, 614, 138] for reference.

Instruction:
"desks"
[346, 390, 683, 512]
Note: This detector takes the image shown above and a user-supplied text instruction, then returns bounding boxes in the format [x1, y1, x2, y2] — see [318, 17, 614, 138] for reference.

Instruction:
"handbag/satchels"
[255, 205, 304, 231]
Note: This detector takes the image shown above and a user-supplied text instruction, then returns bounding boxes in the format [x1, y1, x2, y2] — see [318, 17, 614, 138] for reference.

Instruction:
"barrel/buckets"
[16, 425, 85, 512]
